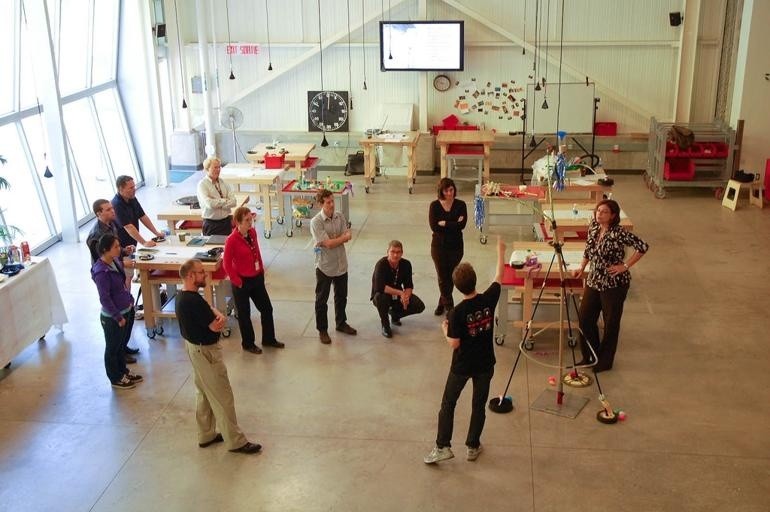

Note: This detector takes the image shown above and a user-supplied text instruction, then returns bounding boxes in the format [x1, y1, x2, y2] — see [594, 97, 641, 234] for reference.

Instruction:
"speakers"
[669, 12, 681, 26]
[154, 22, 166, 38]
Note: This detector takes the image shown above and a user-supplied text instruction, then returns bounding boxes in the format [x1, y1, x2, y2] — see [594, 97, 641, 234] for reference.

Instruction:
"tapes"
[511, 260, 524, 269]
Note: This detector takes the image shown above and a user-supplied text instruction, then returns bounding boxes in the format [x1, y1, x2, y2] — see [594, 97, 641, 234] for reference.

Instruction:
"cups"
[559, 144, 567, 154]
[613, 144, 619, 151]
[568, 145, 572, 150]
[519, 184, 526, 194]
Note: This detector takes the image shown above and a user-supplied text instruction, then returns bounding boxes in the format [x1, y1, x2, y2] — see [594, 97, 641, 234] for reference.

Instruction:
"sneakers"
[466, 445, 482, 461]
[424, 447, 454, 463]
[112, 311, 144, 389]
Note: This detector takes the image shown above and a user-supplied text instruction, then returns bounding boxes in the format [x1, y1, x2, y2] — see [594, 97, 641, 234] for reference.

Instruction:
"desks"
[359, 132, 421, 194]
[0, 255, 70, 371]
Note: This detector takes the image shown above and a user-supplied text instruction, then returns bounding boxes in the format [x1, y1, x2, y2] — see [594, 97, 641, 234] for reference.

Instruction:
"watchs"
[623, 264, 627, 267]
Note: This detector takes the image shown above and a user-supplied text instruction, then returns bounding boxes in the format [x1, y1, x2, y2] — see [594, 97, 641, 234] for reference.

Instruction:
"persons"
[223, 206, 284, 353]
[87, 199, 139, 363]
[198, 158, 236, 236]
[424, 235, 505, 464]
[91, 234, 143, 389]
[571, 201, 648, 372]
[111, 176, 164, 319]
[429, 176, 468, 316]
[369, 240, 426, 338]
[175, 258, 262, 453]
[310, 189, 358, 344]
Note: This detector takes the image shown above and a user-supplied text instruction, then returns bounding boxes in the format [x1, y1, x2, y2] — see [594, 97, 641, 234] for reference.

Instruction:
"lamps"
[317, 1, 393, 147]
[523, 0, 549, 147]
[173, 1, 272, 108]
[23, 2, 52, 179]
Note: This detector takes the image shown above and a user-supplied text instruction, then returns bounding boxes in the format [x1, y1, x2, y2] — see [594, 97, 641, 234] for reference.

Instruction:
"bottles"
[314, 245, 322, 268]
[0, 241, 31, 266]
[530, 252, 537, 268]
[400, 288, 406, 303]
[126, 247, 134, 277]
[179, 232, 185, 241]
[525, 249, 531, 269]
[327, 176, 331, 185]
[753, 169, 760, 184]
[572, 203, 578, 219]
[165, 223, 170, 241]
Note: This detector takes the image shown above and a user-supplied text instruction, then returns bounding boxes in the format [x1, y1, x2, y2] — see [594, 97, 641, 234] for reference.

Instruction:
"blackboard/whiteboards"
[525, 82, 595, 136]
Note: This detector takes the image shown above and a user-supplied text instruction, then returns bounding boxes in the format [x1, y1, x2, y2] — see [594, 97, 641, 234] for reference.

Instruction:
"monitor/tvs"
[378, 19, 465, 71]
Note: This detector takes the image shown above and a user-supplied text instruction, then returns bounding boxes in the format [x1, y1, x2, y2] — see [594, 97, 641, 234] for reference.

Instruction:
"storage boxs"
[596, 122, 616, 136]
[432, 115, 476, 135]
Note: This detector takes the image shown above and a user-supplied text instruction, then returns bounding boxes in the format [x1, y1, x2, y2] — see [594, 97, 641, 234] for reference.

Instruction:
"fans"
[218, 106, 244, 163]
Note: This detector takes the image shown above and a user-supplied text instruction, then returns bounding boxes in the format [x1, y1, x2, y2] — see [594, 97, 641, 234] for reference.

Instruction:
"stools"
[721, 178, 767, 211]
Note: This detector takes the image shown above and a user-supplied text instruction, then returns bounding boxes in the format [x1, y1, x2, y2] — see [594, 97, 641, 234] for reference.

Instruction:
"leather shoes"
[228, 442, 261, 453]
[199, 433, 223, 448]
[243, 305, 444, 354]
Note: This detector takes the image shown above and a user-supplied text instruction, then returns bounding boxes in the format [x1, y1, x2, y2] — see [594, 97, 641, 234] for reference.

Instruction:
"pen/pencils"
[166, 253, 177, 254]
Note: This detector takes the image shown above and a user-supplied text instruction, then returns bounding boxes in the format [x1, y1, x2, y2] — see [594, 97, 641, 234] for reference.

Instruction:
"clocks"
[307, 91, 349, 133]
[433, 75, 450, 92]
